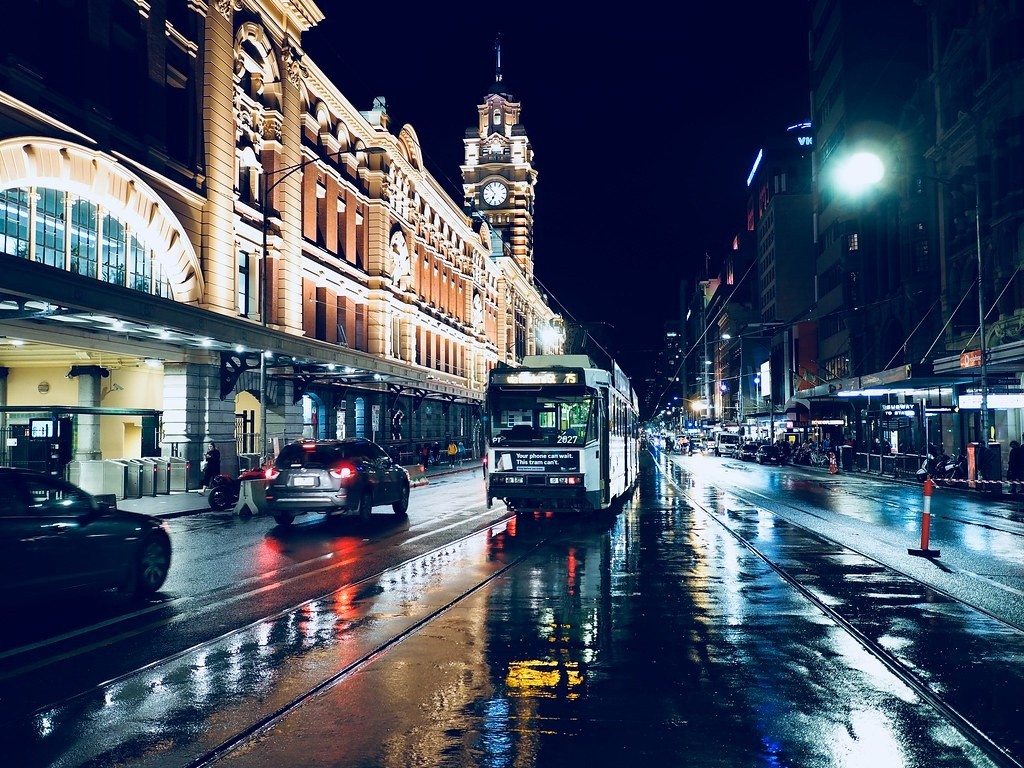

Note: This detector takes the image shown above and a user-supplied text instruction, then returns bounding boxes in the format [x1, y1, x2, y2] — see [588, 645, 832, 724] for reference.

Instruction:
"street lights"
[846, 151, 989, 452]
[705, 359, 725, 423]
[261, 145, 387, 459]
[722, 334, 743, 430]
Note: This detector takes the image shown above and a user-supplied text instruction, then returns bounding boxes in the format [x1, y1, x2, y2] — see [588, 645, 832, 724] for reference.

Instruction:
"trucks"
[714, 431, 741, 457]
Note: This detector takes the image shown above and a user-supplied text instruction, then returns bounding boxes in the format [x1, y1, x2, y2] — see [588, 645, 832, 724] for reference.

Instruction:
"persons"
[641, 434, 705, 457]
[741, 432, 1024, 494]
[197, 442, 221, 496]
[420, 441, 466, 471]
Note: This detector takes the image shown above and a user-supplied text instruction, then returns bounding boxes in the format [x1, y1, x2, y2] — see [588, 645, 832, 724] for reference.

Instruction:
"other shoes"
[1008, 490, 1016, 493]
[449, 465, 451, 468]
[198, 491, 204, 496]
[452, 465, 454, 468]
[1018, 490, 1024, 493]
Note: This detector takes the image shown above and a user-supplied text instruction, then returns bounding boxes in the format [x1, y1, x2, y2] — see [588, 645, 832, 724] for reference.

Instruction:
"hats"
[1011, 441, 1019, 447]
[434, 442, 438, 444]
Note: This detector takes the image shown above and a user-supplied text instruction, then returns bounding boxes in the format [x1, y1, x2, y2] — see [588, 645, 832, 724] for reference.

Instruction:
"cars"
[737, 443, 761, 461]
[676, 433, 715, 450]
[756, 444, 786, 465]
[0, 467, 172, 610]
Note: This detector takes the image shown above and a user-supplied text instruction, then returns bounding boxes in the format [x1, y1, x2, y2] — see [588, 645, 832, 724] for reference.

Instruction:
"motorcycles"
[208, 455, 268, 512]
[915, 442, 968, 488]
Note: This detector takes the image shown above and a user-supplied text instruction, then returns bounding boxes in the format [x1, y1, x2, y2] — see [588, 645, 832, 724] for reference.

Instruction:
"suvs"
[265, 437, 411, 531]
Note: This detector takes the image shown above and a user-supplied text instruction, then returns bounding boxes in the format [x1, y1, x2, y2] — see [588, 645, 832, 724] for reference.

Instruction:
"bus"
[482, 354, 642, 514]
